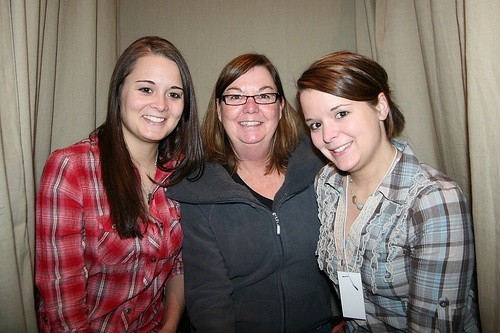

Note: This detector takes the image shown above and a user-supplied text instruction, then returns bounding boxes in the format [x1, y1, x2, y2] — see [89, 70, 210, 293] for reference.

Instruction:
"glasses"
[221, 93, 280, 106]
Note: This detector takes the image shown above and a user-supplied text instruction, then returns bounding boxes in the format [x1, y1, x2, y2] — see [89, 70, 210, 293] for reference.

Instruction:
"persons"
[295, 51, 481, 333]
[164, 53, 345, 333]
[34, 36, 205, 333]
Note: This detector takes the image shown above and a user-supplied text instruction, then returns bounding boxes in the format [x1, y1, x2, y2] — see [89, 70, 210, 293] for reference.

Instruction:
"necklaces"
[125, 137, 159, 201]
[348, 176, 364, 210]
